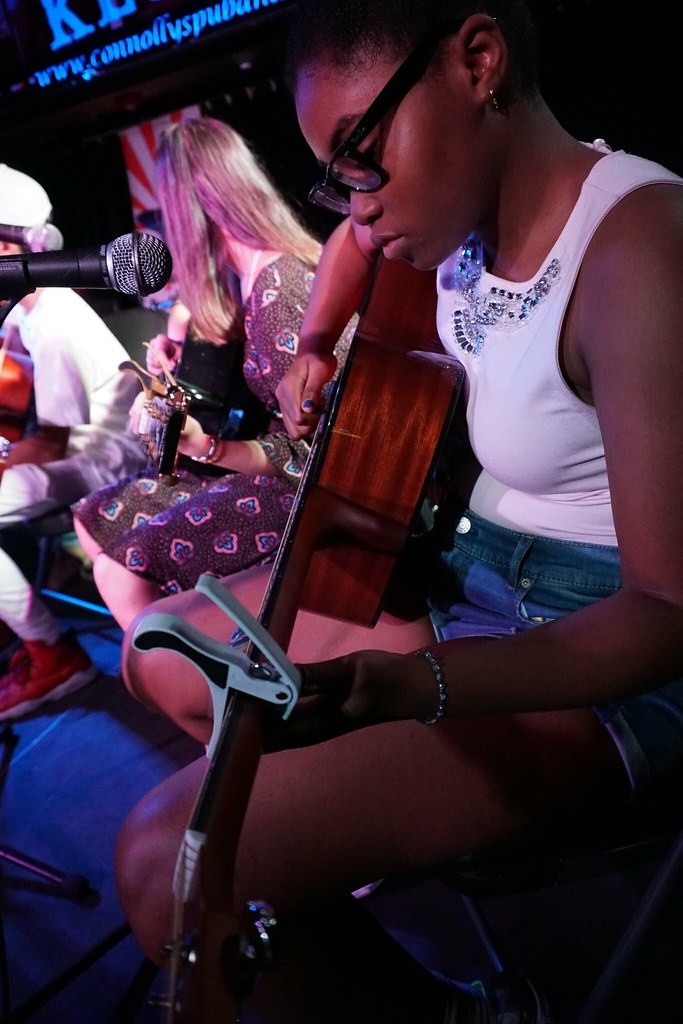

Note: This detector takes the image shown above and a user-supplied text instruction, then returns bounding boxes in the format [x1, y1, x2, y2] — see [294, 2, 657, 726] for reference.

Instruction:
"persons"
[0, 1, 683, 1024]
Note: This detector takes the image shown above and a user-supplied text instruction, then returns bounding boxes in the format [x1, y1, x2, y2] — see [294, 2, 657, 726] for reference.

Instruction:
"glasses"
[307, 10, 503, 217]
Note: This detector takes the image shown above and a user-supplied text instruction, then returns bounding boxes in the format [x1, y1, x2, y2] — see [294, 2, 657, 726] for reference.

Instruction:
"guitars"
[0, 324, 37, 484]
[116, 317, 244, 476]
[127, 251, 466, 1024]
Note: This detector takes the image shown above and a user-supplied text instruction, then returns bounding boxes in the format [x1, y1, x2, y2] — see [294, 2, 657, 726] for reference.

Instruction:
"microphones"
[0, 231, 173, 297]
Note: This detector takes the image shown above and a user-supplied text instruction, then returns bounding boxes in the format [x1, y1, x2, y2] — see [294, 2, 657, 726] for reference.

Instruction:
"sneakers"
[0, 627, 96, 719]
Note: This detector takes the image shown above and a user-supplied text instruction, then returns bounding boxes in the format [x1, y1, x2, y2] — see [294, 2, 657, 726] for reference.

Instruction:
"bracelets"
[416, 650, 445, 725]
[200, 439, 223, 465]
[0, 436, 12, 459]
[191, 434, 216, 464]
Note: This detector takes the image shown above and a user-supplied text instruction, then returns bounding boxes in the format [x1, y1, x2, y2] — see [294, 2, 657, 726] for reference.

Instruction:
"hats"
[0, 163, 52, 226]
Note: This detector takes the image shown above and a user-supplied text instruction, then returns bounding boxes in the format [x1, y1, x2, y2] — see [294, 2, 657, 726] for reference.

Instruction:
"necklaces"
[247, 249, 262, 294]
